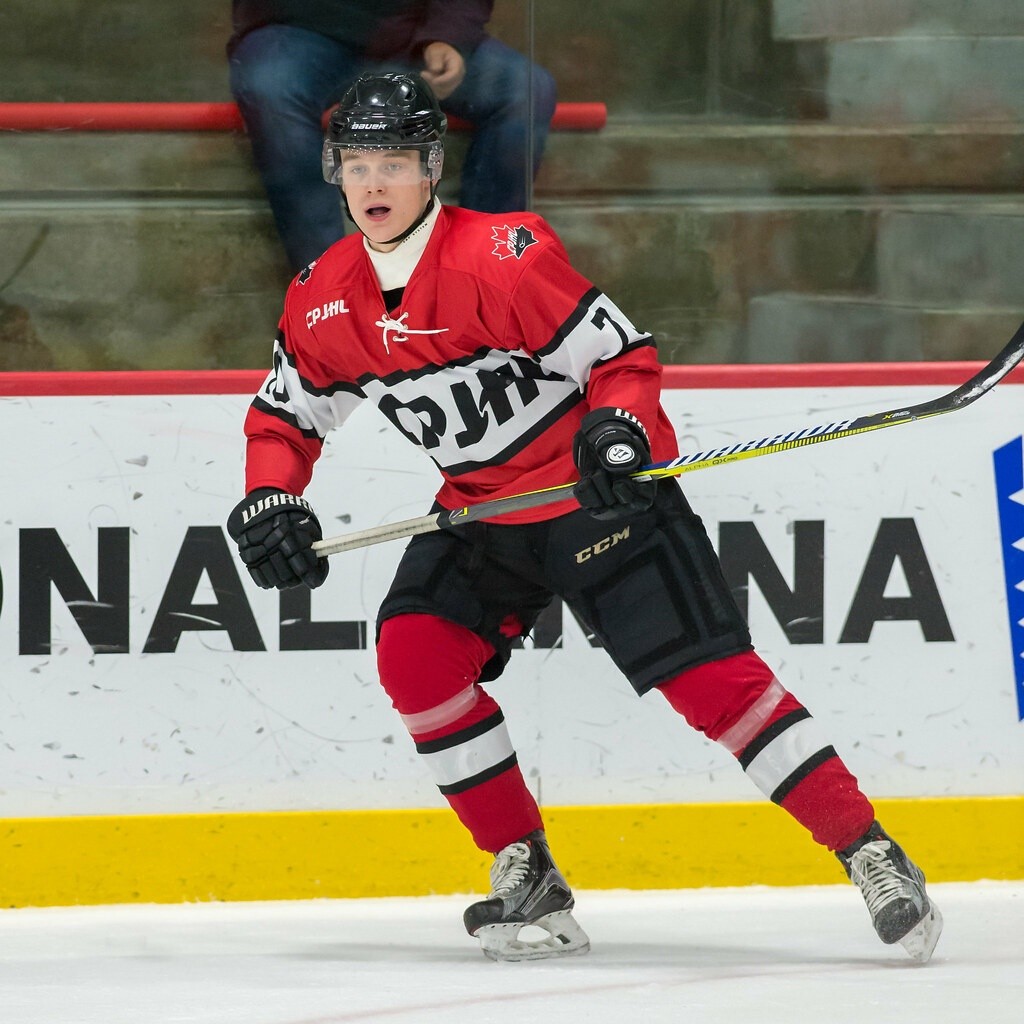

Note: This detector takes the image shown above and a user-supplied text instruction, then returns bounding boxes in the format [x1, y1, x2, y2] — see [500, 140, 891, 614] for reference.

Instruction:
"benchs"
[1, 103, 607, 391]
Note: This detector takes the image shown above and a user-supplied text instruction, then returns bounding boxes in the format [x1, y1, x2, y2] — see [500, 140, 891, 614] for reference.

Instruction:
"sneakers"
[834, 819, 943, 965]
[463, 830, 590, 962]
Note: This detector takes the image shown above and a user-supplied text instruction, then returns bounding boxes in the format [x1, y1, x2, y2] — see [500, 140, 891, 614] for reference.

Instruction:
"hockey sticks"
[308, 317, 1024, 556]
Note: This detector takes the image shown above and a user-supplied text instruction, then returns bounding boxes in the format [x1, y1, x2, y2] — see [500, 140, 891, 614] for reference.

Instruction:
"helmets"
[327, 71, 448, 177]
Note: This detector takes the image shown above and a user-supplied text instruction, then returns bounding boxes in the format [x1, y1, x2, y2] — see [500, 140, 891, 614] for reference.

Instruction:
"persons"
[226, 62, 943, 966]
[225, 0, 560, 279]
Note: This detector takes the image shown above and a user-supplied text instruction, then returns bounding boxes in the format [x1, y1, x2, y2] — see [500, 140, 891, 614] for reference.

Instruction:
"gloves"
[227, 486, 330, 590]
[572, 407, 658, 521]
